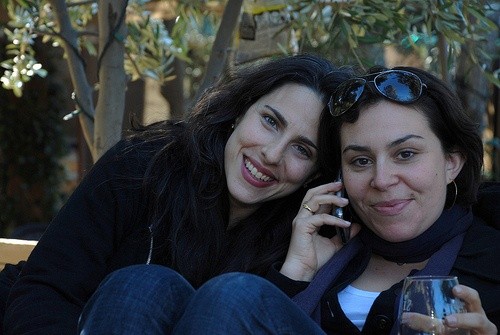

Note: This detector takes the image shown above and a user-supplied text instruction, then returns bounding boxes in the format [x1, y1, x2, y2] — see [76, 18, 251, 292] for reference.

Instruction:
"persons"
[0, 53, 366, 334]
[79, 64, 500, 335]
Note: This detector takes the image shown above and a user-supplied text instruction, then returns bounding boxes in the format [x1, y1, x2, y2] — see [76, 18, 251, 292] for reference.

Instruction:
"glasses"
[327, 70, 427, 117]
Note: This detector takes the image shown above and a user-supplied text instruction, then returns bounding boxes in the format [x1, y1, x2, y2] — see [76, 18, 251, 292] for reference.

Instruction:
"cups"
[398, 275, 471, 335]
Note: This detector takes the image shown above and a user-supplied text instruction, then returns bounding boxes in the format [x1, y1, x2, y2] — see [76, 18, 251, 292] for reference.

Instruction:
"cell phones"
[334, 169, 352, 245]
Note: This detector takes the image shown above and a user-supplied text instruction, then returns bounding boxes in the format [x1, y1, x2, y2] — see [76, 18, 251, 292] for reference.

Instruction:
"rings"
[303, 204, 316, 215]
